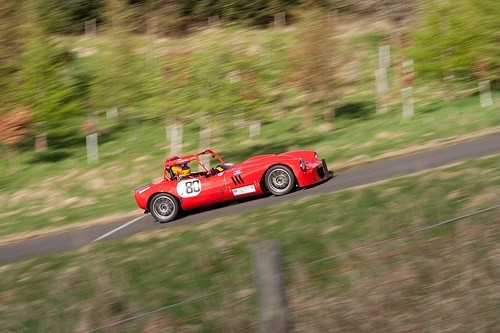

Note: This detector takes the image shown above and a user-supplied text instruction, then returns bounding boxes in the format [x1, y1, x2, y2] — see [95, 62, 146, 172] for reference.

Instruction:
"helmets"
[171, 158, 192, 176]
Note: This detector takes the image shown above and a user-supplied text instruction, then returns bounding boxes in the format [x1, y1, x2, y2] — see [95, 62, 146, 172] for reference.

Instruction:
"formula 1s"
[134, 148, 333, 223]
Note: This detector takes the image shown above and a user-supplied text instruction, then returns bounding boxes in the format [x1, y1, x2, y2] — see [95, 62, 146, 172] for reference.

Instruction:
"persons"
[170, 164, 206, 181]
